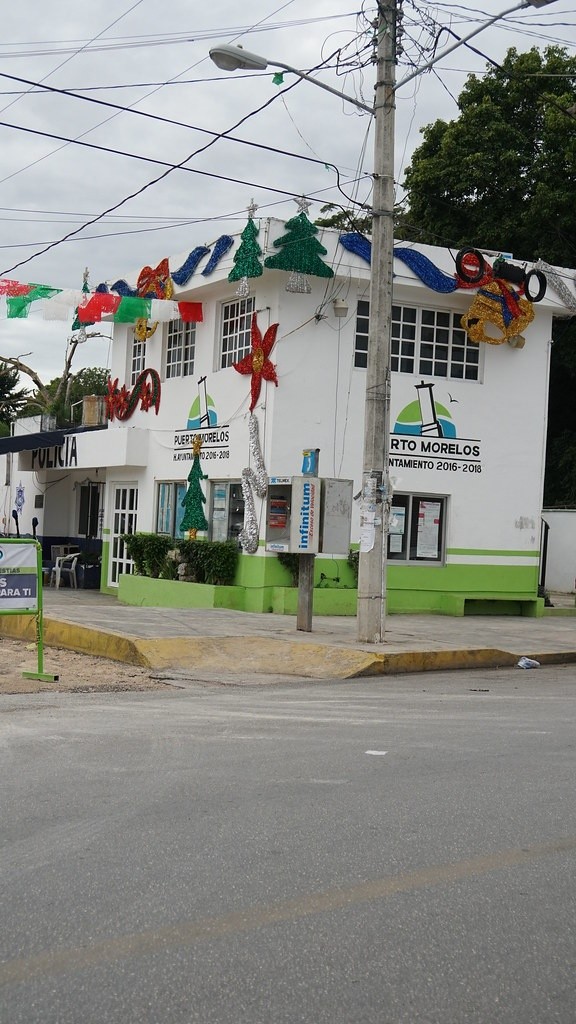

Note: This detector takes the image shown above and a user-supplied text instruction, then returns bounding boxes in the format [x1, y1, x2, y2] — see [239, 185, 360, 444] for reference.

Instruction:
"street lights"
[205, 43, 388, 645]
[352, 0, 565, 645]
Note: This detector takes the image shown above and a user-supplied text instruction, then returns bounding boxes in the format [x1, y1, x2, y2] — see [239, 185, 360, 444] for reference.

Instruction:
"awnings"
[0, 430, 63, 455]
